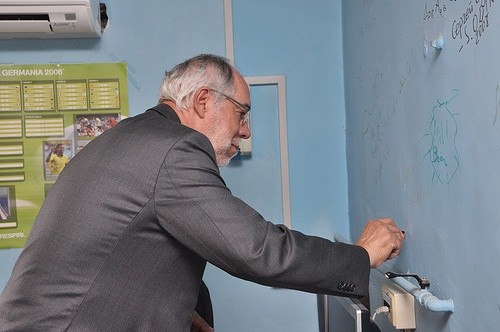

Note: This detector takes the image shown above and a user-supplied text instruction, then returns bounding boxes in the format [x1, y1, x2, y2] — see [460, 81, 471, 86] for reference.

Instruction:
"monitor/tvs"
[324, 294, 370, 332]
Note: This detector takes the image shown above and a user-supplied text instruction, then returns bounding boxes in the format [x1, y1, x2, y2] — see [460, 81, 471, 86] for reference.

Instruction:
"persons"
[77, 116, 117, 137]
[46, 143, 68, 176]
[0, 53, 404, 332]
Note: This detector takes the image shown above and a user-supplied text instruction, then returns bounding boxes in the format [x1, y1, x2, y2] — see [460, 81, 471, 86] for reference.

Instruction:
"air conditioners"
[0, 0, 110, 39]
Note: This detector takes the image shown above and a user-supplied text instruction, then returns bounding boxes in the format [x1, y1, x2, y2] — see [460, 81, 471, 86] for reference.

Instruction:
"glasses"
[210, 88, 250, 119]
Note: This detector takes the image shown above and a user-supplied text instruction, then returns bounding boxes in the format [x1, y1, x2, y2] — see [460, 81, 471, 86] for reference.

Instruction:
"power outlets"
[381, 284, 416, 330]
